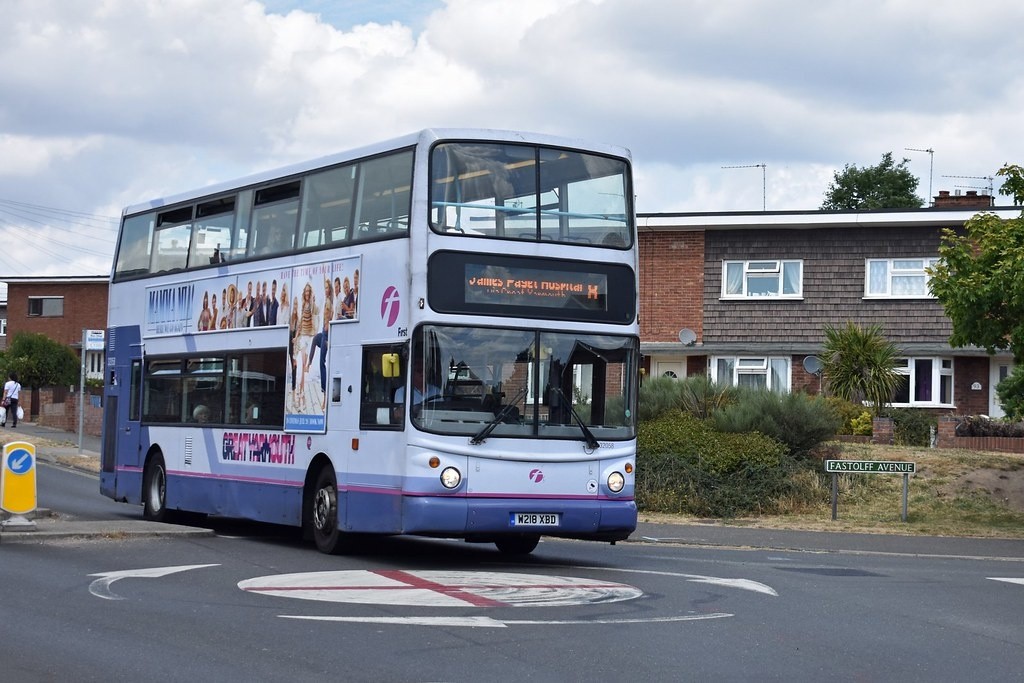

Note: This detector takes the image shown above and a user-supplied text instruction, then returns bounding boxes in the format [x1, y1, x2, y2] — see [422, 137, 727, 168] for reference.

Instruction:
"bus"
[99, 127, 643, 560]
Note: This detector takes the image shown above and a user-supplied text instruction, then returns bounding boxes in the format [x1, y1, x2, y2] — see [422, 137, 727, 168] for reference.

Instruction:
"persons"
[192, 392, 263, 425]
[0, 373, 22, 428]
[197, 270, 360, 412]
[394, 358, 445, 424]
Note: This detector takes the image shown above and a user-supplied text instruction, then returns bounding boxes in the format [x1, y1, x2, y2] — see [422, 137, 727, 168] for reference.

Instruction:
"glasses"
[414, 368, 422, 374]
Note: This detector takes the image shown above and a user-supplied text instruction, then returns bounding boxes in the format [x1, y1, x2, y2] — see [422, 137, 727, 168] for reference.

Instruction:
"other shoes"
[11, 426, 16, 428]
[303, 403, 305, 410]
[0, 425, 5, 427]
[298, 406, 301, 412]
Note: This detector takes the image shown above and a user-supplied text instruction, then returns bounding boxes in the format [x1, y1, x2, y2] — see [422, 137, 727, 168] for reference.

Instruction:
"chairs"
[497, 404, 519, 424]
[358, 220, 593, 245]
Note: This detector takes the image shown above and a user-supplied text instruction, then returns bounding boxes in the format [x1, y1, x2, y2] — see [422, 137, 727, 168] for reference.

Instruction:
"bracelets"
[2, 400, 5, 401]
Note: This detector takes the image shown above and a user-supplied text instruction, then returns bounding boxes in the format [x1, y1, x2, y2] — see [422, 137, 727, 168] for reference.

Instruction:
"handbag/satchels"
[3, 398, 11, 406]
[0, 407, 6, 423]
[16, 406, 24, 419]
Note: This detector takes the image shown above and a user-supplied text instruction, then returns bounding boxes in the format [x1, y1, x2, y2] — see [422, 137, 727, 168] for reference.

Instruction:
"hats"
[227, 284, 238, 306]
